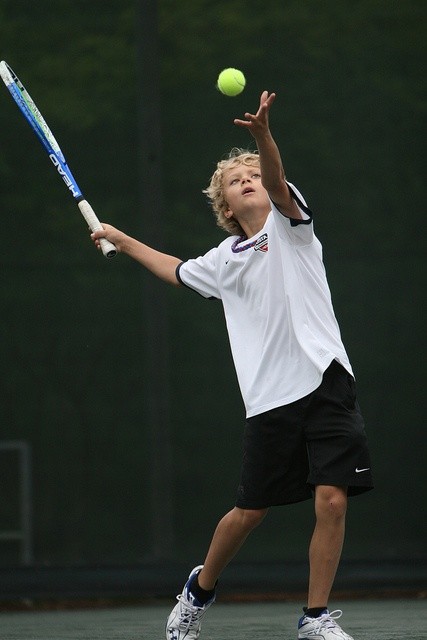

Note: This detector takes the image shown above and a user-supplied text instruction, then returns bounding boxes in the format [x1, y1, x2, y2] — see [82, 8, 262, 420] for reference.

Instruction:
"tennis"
[217, 67, 246, 97]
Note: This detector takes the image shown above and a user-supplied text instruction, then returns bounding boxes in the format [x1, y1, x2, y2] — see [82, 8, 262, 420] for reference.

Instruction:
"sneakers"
[165, 565, 218, 640]
[298, 607, 354, 640]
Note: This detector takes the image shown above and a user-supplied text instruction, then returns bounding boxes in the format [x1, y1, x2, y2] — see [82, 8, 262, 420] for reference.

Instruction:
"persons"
[88, 88, 378, 640]
